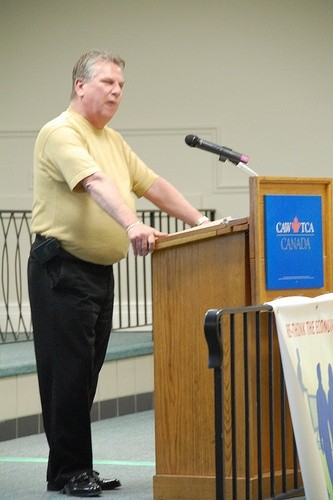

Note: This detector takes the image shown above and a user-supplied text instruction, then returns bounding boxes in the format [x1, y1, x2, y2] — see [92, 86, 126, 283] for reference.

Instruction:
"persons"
[27, 50, 211, 496]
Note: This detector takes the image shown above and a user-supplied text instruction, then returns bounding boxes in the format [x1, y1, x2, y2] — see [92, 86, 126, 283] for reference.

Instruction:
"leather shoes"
[91, 471, 121, 489]
[61, 472, 102, 496]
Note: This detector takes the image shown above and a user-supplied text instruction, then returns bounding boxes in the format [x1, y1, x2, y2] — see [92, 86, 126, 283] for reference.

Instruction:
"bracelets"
[125, 220, 143, 234]
[192, 216, 210, 226]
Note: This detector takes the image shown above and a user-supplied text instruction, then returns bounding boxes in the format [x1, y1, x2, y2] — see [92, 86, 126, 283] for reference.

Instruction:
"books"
[168, 216, 231, 236]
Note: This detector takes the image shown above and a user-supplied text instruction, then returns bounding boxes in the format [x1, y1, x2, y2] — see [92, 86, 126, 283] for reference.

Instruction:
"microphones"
[185, 134, 249, 163]
